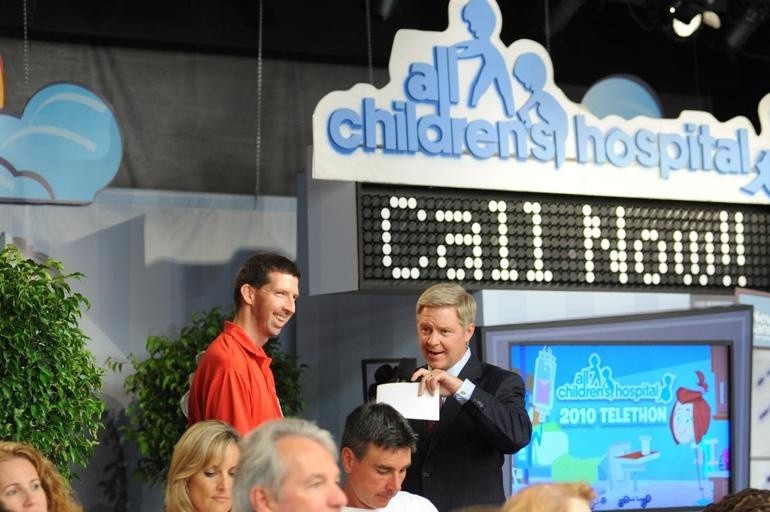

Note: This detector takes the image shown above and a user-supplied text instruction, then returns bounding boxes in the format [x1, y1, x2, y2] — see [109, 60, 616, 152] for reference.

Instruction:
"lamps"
[665, 0, 723, 44]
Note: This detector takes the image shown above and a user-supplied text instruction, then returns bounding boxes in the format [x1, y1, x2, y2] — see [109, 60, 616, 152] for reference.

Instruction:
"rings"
[431, 375, 434, 377]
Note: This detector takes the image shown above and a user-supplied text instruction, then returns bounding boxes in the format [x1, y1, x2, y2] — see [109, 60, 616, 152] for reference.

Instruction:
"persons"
[340, 402, 439, 512]
[164, 419, 244, 511]
[375, 282, 532, 511]
[233, 418, 348, 512]
[0, 438, 84, 512]
[505, 481, 598, 511]
[188, 254, 300, 434]
